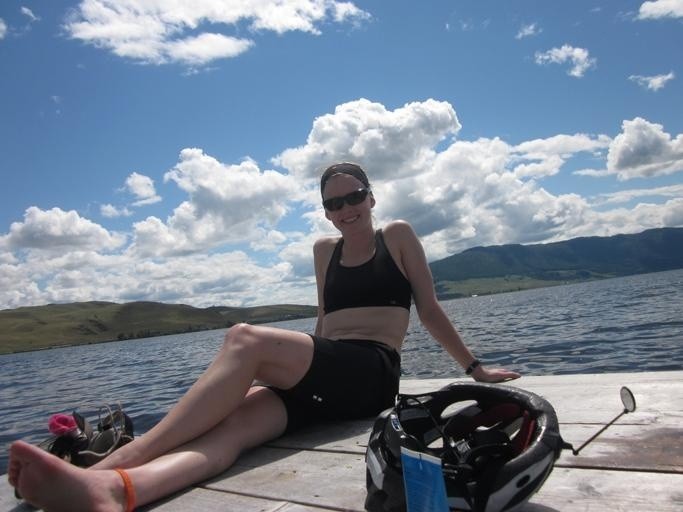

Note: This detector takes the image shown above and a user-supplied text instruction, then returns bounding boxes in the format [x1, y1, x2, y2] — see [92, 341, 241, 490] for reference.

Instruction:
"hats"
[321, 164, 370, 194]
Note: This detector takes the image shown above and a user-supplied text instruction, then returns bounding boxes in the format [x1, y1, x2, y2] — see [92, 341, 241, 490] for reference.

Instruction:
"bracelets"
[466, 359, 480, 375]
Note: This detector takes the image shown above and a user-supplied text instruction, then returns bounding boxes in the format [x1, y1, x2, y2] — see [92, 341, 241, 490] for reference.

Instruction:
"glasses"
[323, 187, 371, 211]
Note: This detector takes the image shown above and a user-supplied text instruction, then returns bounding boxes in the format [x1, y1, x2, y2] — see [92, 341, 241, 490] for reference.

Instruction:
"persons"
[7, 161, 521, 512]
